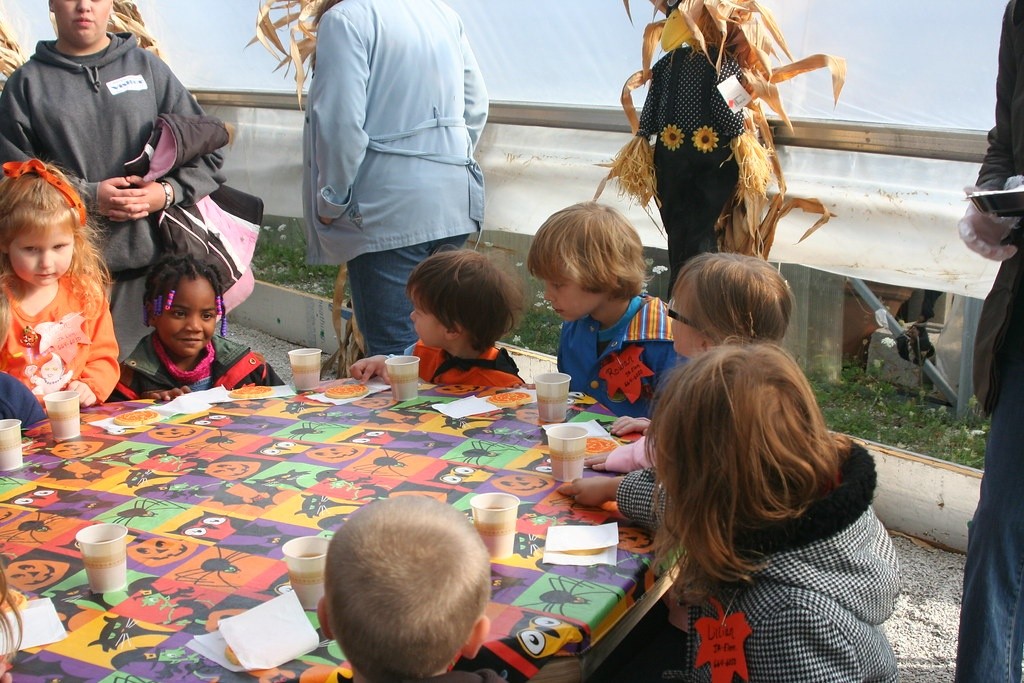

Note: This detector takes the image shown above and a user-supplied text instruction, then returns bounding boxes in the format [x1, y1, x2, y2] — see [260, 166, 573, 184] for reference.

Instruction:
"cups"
[282, 536, 329, 610]
[76, 523, 128, 593]
[546, 426, 589, 482]
[0, 419, 23, 472]
[385, 356, 420, 401]
[469, 492, 521, 559]
[43, 391, 81, 440]
[534, 373, 571, 423]
[288, 348, 322, 391]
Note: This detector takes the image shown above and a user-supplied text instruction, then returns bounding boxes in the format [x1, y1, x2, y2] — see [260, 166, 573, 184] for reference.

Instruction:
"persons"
[318, 496, 509, 682]
[0, 288, 45, 428]
[556, 343, 899, 683]
[526, 203, 690, 415]
[0, 0, 226, 358]
[302, 0, 488, 353]
[952, 0, 1024, 683]
[582, 253, 791, 473]
[106, 253, 286, 403]
[350, 249, 527, 385]
[603, 0, 773, 302]
[0, 159, 121, 417]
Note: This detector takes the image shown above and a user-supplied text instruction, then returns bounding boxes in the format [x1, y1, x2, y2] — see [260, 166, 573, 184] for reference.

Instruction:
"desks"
[0, 376, 680, 683]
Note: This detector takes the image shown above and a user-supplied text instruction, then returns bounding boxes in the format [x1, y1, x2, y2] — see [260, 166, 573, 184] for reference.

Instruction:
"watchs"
[161, 182, 171, 210]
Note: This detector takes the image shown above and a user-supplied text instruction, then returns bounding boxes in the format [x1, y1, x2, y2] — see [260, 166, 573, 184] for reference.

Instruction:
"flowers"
[658, 123, 686, 153]
[689, 126, 721, 153]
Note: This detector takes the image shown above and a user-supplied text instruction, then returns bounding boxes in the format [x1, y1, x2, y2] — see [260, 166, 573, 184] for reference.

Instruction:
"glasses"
[666, 298, 721, 345]
